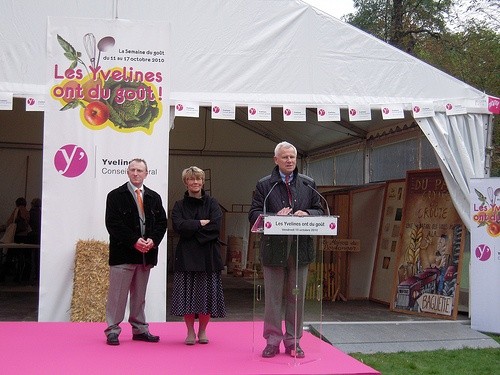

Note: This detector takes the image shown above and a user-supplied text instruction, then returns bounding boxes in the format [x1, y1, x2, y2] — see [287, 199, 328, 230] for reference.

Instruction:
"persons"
[247, 141, 325, 358]
[105, 158, 167, 345]
[170, 166, 226, 344]
[5, 197, 32, 242]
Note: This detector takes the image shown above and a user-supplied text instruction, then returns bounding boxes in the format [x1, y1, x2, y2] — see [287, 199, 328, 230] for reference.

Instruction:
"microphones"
[264, 179, 283, 214]
[303, 182, 330, 216]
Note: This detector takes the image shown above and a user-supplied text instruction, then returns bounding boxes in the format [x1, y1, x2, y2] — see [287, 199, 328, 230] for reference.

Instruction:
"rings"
[143, 246, 144, 249]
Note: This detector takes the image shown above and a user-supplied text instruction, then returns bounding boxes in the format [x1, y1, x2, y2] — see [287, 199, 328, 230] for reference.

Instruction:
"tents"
[0, 0, 500, 322]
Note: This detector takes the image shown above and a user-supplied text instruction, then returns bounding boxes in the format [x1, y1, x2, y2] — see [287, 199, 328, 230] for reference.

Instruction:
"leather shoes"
[262, 343, 279, 356]
[287, 343, 304, 357]
[133, 331, 160, 342]
[107, 334, 119, 345]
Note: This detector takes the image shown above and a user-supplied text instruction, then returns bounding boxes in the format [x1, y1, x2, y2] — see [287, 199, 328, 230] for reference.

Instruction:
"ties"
[135, 188, 144, 216]
[285, 175, 292, 206]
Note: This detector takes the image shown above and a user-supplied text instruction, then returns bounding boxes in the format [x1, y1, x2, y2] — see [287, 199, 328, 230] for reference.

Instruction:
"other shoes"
[199, 330, 208, 343]
[185, 334, 196, 345]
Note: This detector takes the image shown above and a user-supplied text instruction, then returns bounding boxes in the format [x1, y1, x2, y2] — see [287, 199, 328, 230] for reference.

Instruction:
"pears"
[61, 73, 102, 103]
[476, 206, 499, 225]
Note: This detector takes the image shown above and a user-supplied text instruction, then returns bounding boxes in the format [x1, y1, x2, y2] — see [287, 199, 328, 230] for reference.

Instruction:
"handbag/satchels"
[0, 223, 17, 244]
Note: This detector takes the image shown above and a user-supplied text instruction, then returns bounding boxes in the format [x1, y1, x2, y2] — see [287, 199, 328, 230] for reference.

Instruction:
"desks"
[0, 244, 40, 248]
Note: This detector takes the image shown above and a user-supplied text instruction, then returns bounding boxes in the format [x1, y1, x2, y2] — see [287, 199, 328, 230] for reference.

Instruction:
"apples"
[84, 101, 109, 126]
[488, 222, 500, 235]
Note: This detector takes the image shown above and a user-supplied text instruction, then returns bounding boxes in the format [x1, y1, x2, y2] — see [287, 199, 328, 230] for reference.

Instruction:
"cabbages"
[103, 76, 159, 129]
